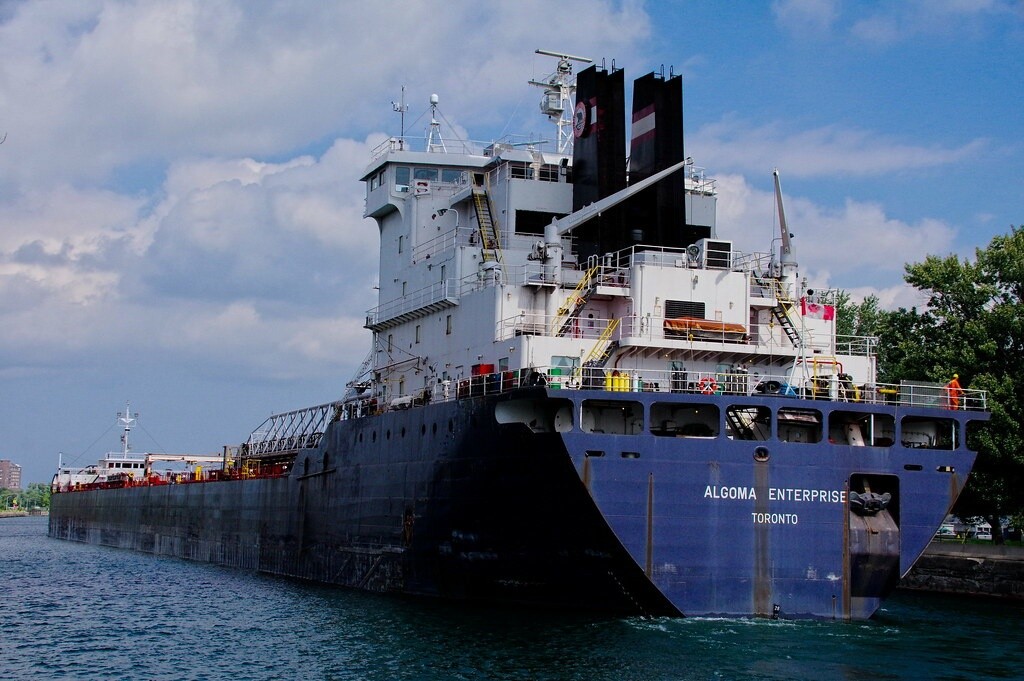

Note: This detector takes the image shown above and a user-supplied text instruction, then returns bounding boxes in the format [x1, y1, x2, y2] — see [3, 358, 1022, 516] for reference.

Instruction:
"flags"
[805, 303, 835, 320]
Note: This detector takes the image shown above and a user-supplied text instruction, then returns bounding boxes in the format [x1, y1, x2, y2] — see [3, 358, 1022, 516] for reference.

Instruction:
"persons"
[943, 372, 965, 411]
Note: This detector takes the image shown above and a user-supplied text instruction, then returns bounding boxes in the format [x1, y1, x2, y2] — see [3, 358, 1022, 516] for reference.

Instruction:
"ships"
[49, 48, 991, 617]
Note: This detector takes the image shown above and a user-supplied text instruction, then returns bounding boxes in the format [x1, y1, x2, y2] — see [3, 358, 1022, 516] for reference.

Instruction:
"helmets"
[953, 374, 959, 378]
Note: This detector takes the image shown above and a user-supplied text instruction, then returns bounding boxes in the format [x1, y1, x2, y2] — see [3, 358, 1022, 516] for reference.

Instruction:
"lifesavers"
[698, 377, 717, 395]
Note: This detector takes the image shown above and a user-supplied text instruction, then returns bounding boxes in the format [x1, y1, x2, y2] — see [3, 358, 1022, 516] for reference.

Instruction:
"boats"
[935, 521, 993, 540]
